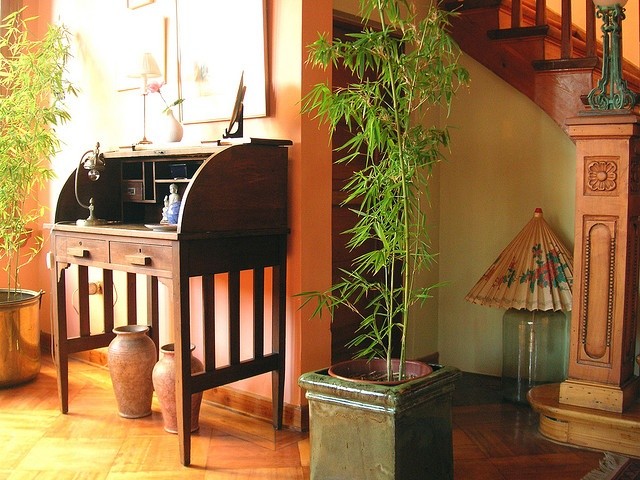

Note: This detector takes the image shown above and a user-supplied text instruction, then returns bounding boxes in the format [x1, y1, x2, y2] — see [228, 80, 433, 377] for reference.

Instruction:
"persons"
[162, 184, 181, 221]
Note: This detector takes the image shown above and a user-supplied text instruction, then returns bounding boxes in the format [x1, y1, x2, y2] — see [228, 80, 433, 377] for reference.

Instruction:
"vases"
[163, 108, 184, 143]
[151, 342, 204, 436]
[105, 321, 155, 421]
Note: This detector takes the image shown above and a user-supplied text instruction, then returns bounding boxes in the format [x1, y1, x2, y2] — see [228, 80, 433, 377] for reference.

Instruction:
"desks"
[41, 137, 293, 467]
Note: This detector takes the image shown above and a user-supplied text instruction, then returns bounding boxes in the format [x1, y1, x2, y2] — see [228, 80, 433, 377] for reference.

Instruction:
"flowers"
[147, 81, 187, 112]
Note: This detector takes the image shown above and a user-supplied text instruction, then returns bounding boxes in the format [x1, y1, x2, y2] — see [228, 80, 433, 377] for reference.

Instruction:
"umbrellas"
[463, 208, 573, 339]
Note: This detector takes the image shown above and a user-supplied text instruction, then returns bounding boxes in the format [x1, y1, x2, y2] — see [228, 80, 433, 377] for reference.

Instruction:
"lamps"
[126, 53, 162, 143]
[74, 140, 108, 226]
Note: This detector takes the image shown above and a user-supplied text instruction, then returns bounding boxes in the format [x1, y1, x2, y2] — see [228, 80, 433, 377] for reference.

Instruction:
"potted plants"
[1, 5, 79, 394]
[297, 1, 473, 479]
